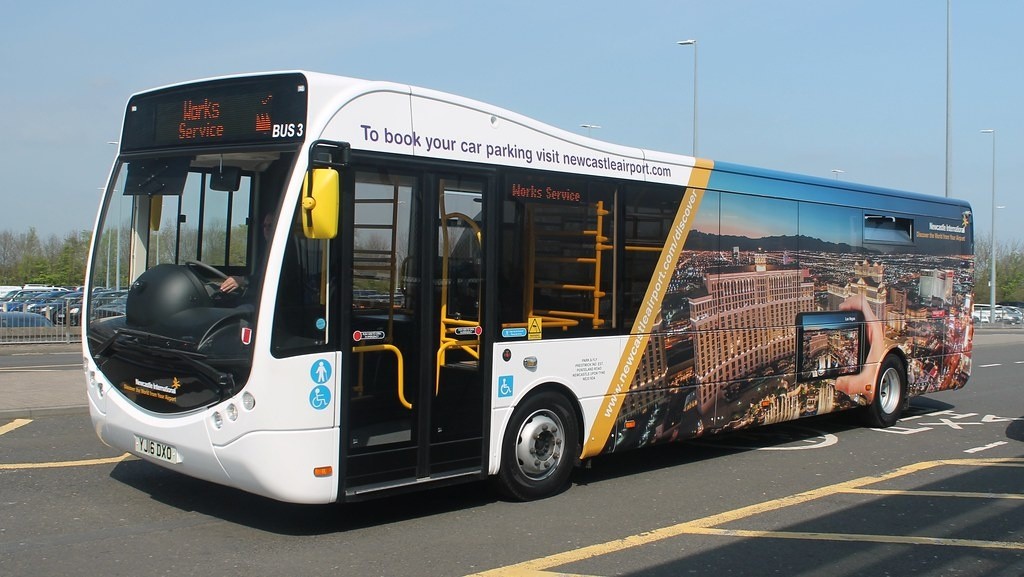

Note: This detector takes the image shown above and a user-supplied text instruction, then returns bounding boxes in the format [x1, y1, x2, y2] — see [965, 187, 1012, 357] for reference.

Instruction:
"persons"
[218, 213, 305, 313]
[836, 294, 889, 406]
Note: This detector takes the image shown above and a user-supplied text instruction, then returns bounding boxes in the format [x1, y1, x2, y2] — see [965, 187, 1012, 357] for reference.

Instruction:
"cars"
[972, 302, 1024, 325]
[0, 284, 130, 330]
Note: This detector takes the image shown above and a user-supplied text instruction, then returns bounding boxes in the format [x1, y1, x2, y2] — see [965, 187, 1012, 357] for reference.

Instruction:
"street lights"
[832, 169, 844, 181]
[579, 123, 601, 141]
[678, 39, 699, 158]
[980, 129, 999, 325]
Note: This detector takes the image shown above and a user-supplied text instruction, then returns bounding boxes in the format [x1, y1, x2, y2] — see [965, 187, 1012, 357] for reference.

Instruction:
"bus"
[81, 69, 972, 507]
